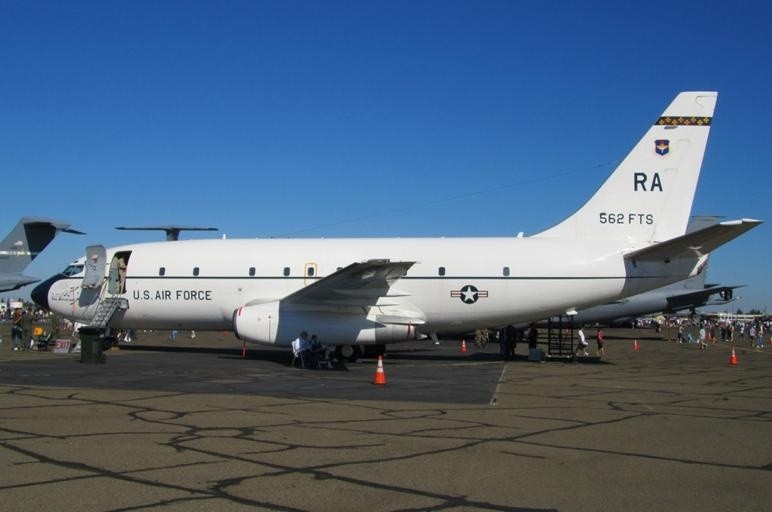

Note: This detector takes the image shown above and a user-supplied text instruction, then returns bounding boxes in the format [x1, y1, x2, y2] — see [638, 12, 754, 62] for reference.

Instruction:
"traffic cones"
[461, 339, 467, 352]
[632, 337, 639, 352]
[369, 355, 386, 386]
[729, 347, 738, 366]
[223, 330, 229, 336]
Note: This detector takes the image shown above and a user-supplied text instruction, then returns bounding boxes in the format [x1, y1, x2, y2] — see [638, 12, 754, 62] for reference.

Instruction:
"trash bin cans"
[78, 327, 105, 365]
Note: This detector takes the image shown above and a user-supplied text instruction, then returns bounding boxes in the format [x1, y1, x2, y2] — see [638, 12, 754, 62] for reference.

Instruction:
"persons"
[310, 333, 333, 370]
[596, 328, 608, 357]
[168, 330, 179, 341]
[1, 304, 138, 352]
[294, 331, 314, 370]
[118, 258, 128, 293]
[575, 325, 590, 357]
[634, 313, 772, 352]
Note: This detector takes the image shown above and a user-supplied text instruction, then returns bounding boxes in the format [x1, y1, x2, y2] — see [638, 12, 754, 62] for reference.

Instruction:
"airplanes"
[31, 88, 765, 375]
[0, 215, 89, 295]
[487, 215, 746, 342]
[109, 225, 219, 241]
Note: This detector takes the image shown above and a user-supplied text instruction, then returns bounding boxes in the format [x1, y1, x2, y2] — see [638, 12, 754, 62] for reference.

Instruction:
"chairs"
[312, 344, 332, 369]
[25, 331, 54, 351]
[291, 342, 322, 370]
